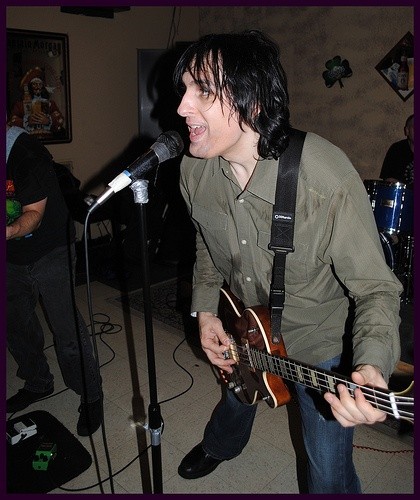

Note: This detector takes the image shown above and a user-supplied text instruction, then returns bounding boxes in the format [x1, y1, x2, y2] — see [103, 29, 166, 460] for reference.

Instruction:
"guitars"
[210, 287, 416, 426]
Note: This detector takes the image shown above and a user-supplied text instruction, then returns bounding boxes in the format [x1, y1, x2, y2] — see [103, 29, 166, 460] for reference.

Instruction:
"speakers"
[136, 48, 196, 174]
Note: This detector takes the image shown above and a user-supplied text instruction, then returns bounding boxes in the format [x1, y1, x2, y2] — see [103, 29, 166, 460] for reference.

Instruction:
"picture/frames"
[375, 31, 414, 101]
[136, 49, 190, 153]
[6, 28, 73, 144]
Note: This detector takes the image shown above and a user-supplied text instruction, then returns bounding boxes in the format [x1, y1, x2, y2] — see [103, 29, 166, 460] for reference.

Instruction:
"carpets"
[106, 275, 201, 346]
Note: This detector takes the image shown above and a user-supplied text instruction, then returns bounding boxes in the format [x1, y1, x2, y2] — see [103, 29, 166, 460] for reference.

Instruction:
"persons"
[6, 125, 103, 437]
[380, 114, 416, 186]
[172, 31, 404, 494]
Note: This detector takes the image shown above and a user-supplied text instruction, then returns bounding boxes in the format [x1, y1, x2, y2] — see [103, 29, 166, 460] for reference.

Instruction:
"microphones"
[88, 130, 185, 214]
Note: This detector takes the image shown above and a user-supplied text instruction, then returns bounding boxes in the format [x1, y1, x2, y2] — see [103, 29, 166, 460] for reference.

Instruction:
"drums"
[362, 177, 415, 235]
[375, 228, 406, 273]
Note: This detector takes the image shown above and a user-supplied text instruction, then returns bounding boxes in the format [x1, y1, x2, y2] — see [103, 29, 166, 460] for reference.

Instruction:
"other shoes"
[77, 391, 103, 436]
[7, 381, 54, 413]
[178, 443, 223, 479]
